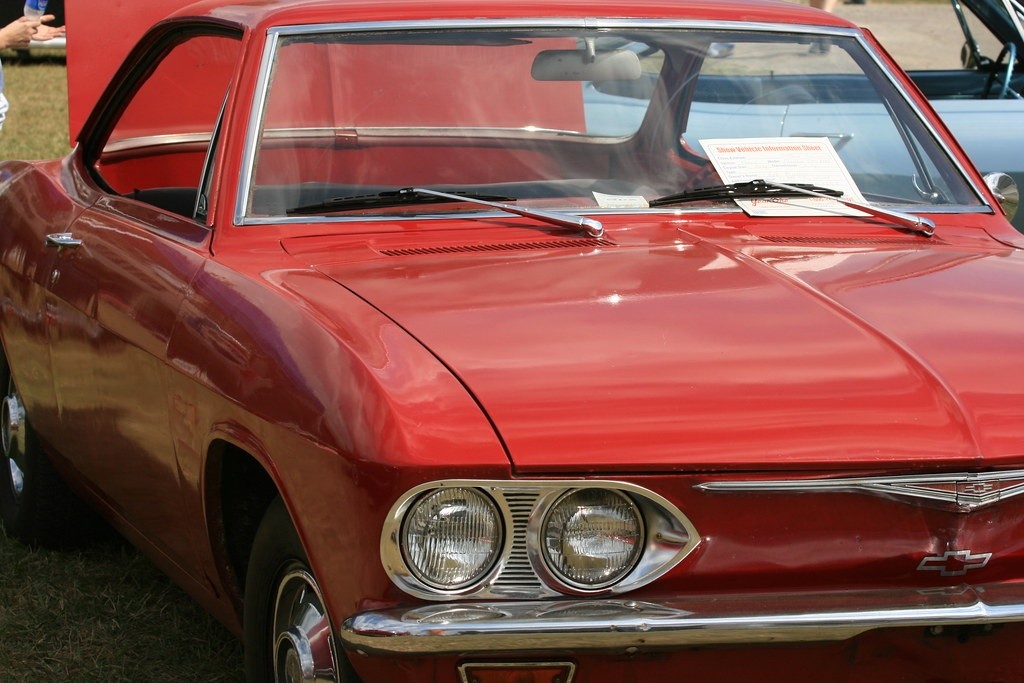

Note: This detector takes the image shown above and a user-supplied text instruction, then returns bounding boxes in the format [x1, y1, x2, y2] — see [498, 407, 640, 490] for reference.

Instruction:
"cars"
[579, 1, 1022, 236]
[0, 2, 1024, 683]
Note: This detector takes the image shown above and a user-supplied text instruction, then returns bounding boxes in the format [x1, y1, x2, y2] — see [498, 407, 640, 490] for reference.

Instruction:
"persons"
[0, 0, 66, 129]
[807, 1, 838, 56]
